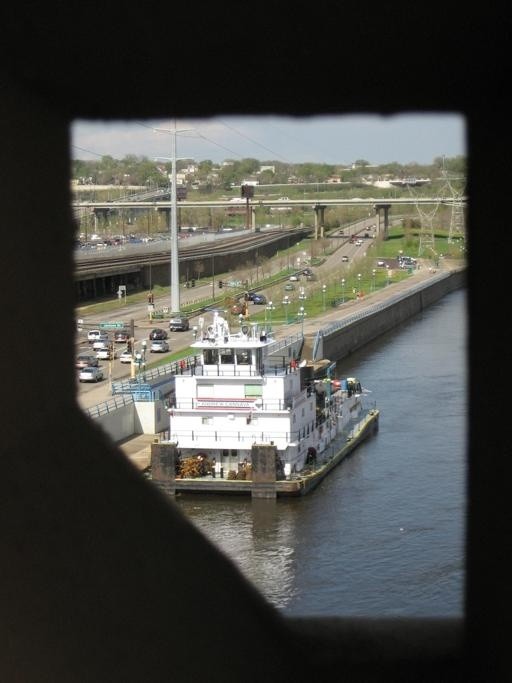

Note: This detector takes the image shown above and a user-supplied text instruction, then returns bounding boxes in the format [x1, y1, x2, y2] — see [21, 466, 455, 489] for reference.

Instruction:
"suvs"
[168, 316, 188, 331]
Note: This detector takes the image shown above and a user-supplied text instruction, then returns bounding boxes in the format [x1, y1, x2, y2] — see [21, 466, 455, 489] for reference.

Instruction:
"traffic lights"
[218, 281, 222, 288]
[191, 278, 195, 287]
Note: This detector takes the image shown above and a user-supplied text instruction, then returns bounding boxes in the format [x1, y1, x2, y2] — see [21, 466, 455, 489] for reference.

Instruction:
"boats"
[142, 308, 379, 497]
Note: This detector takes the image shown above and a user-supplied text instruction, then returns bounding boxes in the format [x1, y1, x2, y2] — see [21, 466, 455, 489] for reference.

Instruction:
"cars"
[399, 256, 416, 269]
[149, 329, 168, 353]
[246, 292, 266, 304]
[339, 230, 343, 233]
[121, 348, 140, 362]
[351, 234, 363, 247]
[115, 329, 129, 344]
[285, 268, 315, 290]
[223, 295, 243, 314]
[364, 224, 376, 238]
[343, 255, 348, 262]
[75, 354, 102, 383]
[88, 328, 113, 360]
